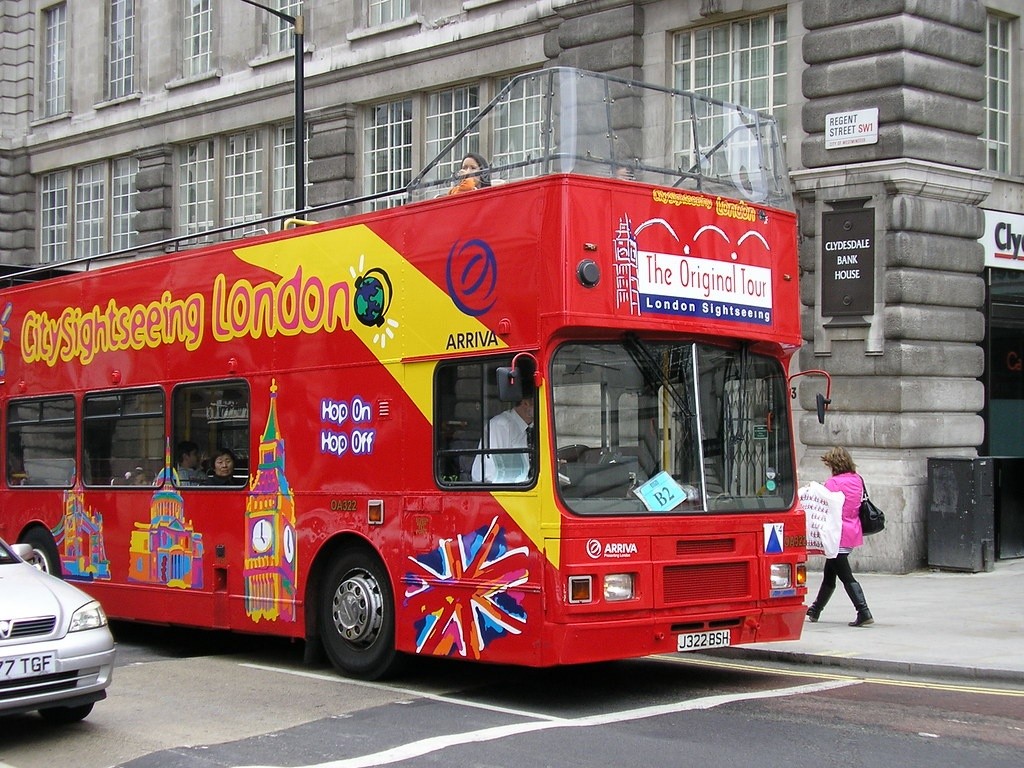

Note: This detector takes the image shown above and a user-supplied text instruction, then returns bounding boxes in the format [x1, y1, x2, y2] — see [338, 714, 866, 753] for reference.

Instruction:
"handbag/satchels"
[798, 481, 845, 559]
[859, 474, 885, 536]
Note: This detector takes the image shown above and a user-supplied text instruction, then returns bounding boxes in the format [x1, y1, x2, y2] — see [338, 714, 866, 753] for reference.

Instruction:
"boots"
[806, 584, 835, 622]
[845, 582, 874, 626]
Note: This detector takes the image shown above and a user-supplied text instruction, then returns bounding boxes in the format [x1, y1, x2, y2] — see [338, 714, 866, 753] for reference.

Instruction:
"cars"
[0, 536, 117, 726]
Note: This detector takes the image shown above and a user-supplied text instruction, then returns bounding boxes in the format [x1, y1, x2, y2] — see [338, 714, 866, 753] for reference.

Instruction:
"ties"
[526, 427, 534, 465]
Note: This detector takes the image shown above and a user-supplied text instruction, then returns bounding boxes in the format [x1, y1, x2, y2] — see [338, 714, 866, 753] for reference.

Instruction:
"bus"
[0, 65, 837, 685]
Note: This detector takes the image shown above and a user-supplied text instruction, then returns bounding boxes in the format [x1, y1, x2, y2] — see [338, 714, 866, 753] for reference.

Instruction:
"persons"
[200, 447, 243, 485]
[449, 153, 491, 196]
[174, 441, 208, 486]
[471, 389, 571, 487]
[127, 469, 148, 485]
[806, 447, 874, 627]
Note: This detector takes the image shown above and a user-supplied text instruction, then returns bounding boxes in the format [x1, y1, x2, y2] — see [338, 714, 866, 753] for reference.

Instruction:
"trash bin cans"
[926, 456, 996, 574]
